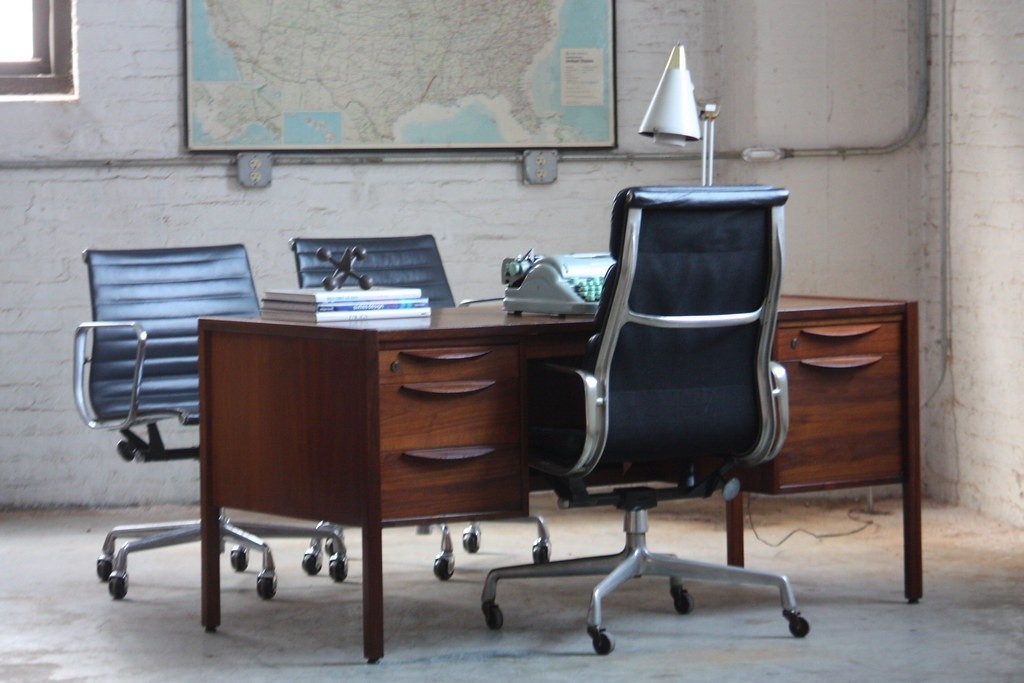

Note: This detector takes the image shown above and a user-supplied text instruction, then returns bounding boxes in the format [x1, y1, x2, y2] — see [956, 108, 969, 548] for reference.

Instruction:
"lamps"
[637, 39, 720, 186]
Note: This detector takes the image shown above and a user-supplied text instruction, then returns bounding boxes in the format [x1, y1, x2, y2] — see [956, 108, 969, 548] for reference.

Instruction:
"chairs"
[74, 244, 348, 599]
[291, 235, 551, 582]
[479, 186, 810, 659]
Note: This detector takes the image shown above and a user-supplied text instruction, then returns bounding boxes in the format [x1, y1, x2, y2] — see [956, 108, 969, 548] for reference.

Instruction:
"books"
[261, 286, 431, 322]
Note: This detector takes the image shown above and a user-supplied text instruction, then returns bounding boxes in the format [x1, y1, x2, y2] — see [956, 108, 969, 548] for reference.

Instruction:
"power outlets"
[237, 150, 272, 188]
[521, 149, 559, 183]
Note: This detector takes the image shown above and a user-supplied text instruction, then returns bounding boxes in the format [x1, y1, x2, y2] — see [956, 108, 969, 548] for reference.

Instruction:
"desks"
[199, 295, 926, 667]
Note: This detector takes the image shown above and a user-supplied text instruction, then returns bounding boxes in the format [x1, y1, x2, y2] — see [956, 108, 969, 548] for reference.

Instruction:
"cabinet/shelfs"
[377, 340, 530, 528]
[740, 316, 905, 496]
